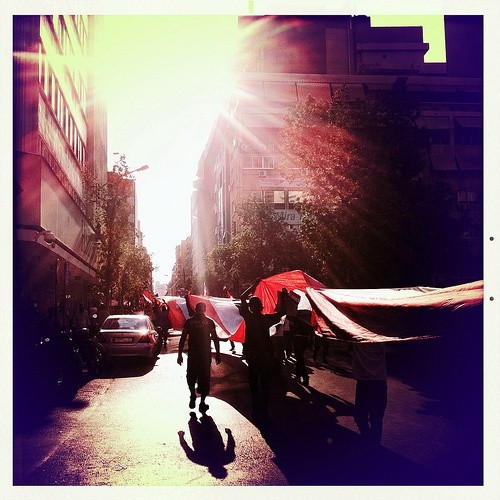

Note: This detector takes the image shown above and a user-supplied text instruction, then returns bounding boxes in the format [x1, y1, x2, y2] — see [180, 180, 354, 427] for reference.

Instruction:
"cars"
[98, 314, 160, 359]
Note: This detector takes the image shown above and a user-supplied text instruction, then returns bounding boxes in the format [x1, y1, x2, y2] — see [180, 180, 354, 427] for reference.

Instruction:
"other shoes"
[199, 402, 209, 413]
[189, 396, 197, 409]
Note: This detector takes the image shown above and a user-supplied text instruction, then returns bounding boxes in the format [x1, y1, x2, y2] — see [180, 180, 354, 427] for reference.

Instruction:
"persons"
[312, 323, 329, 365]
[153, 298, 171, 346]
[240, 278, 287, 421]
[48, 301, 111, 374]
[351, 341, 388, 443]
[285, 310, 313, 386]
[178, 302, 221, 410]
